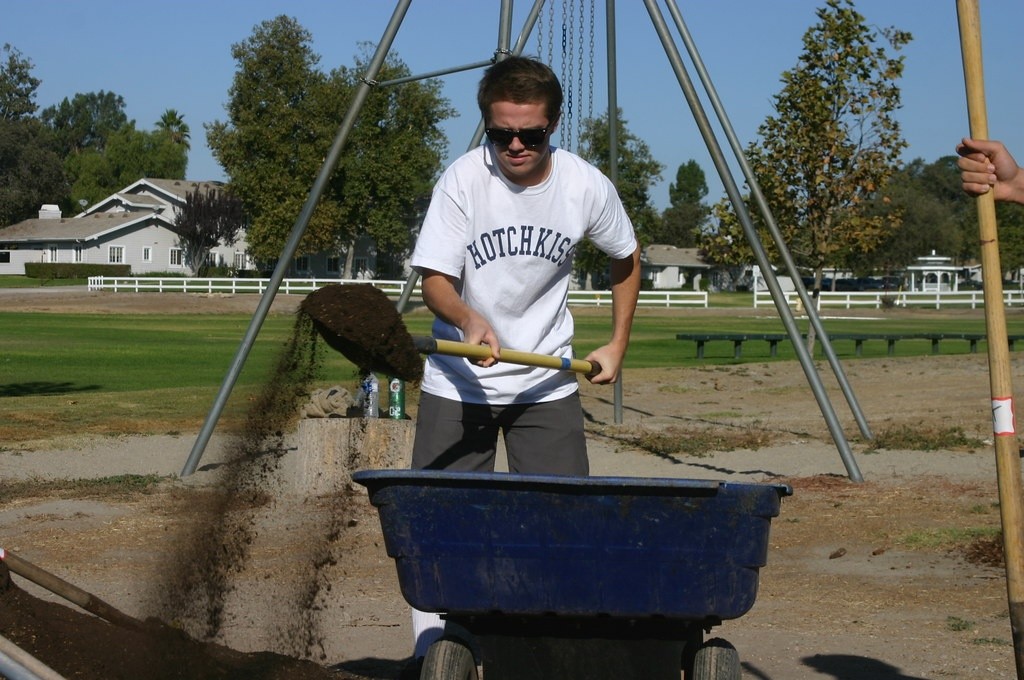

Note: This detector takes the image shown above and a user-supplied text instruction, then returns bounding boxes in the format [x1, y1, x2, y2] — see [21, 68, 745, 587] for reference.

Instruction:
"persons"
[955, 137, 1024, 205]
[407, 55, 641, 680]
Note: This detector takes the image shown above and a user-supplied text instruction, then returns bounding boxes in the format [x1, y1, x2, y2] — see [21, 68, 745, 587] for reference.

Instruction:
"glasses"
[486, 115, 557, 149]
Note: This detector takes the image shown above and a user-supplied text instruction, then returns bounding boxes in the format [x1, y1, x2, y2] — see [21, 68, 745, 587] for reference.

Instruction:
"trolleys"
[350, 470, 794, 680]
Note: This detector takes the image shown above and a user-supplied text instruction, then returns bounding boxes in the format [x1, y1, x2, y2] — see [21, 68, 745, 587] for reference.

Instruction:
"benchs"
[676, 332, 1024, 360]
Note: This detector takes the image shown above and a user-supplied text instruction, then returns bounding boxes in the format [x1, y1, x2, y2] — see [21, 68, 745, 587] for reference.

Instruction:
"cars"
[799, 275, 903, 291]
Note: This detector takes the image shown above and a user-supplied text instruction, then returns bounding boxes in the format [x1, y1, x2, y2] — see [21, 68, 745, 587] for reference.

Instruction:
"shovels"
[304, 311, 602, 383]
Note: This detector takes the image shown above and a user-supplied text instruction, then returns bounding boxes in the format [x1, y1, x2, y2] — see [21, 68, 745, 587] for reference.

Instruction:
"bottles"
[387, 377, 406, 420]
[361, 372, 380, 418]
